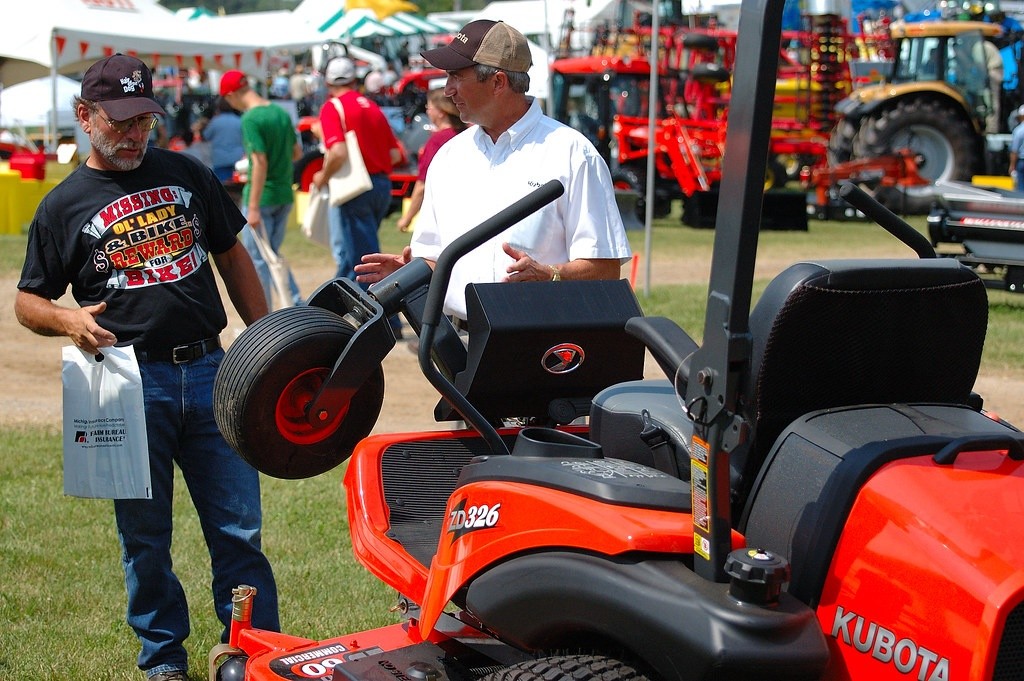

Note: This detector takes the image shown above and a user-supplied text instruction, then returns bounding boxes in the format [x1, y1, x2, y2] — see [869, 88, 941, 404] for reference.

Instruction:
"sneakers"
[148, 669, 189, 681]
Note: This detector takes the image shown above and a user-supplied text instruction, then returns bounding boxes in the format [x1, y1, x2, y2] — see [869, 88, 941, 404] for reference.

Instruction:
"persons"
[312, 57, 404, 340]
[14, 52, 280, 681]
[269, 63, 313, 117]
[397, 87, 473, 233]
[1008, 105, 1024, 190]
[214, 71, 305, 312]
[152, 69, 243, 181]
[353, 18, 632, 430]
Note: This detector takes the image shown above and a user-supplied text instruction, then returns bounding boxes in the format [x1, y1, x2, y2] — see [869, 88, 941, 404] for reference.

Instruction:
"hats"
[420, 20, 532, 73]
[81, 52, 167, 121]
[219, 70, 247, 97]
[324, 57, 356, 85]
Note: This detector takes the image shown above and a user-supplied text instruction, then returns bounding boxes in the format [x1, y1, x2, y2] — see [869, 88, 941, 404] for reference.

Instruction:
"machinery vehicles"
[545, 0, 1024, 294]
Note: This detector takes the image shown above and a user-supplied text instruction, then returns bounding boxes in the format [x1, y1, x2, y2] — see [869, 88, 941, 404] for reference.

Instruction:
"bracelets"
[548, 265, 560, 281]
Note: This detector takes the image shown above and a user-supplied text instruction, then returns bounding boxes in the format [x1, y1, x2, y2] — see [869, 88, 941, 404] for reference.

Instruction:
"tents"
[318, 2, 460, 73]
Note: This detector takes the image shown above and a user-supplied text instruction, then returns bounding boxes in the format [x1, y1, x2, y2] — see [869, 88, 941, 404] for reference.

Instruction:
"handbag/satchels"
[62, 344, 152, 500]
[300, 181, 329, 245]
[249, 221, 304, 308]
[324, 97, 374, 207]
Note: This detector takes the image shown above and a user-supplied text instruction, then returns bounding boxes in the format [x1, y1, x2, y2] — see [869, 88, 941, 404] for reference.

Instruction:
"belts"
[458, 319, 469, 332]
[136, 336, 220, 364]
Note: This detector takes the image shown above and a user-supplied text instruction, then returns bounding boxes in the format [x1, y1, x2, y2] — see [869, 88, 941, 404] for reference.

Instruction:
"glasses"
[88, 106, 158, 133]
[424, 103, 433, 109]
